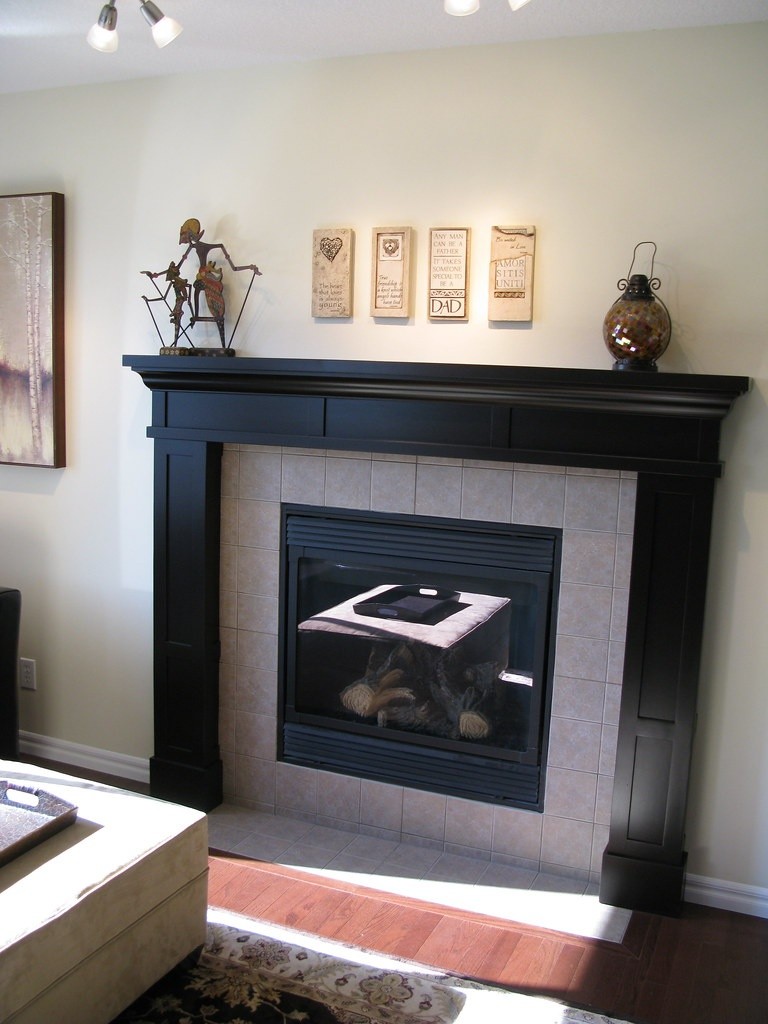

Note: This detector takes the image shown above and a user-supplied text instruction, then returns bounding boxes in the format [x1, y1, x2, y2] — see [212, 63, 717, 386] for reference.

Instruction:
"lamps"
[444, 0, 531, 17]
[86, 0, 182, 54]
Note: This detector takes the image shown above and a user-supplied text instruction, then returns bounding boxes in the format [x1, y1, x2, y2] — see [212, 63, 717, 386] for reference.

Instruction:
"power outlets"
[19, 657, 37, 689]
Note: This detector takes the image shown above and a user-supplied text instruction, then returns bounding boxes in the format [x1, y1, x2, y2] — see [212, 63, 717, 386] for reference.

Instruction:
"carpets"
[106, 905, 639, 1024]
